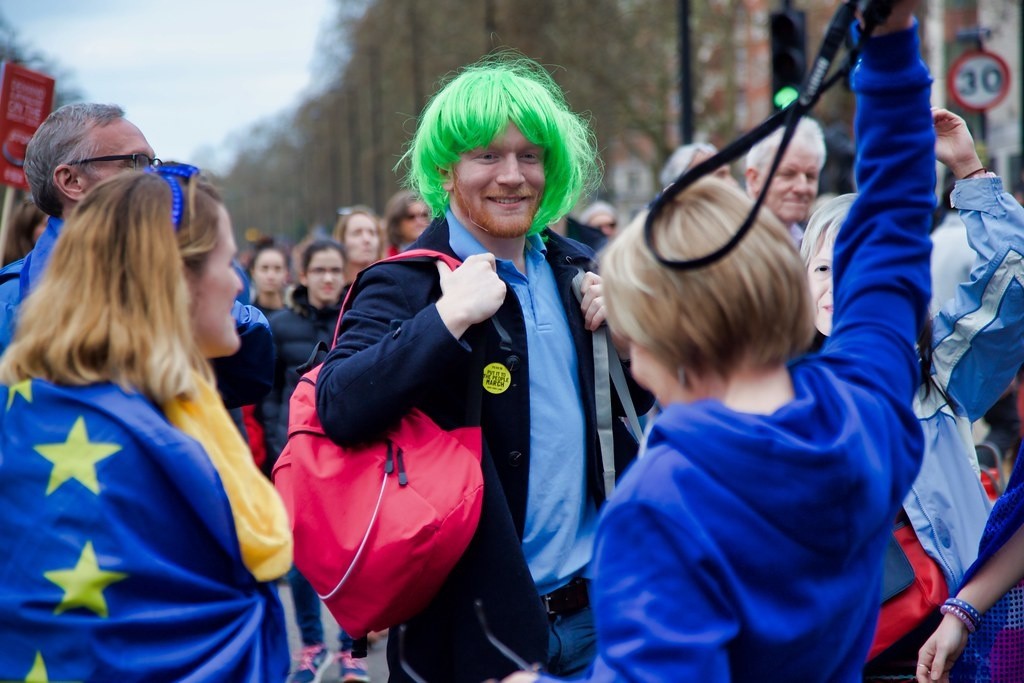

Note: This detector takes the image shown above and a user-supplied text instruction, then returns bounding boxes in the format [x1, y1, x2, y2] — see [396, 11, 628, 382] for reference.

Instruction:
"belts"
[543, 581, 593, 628]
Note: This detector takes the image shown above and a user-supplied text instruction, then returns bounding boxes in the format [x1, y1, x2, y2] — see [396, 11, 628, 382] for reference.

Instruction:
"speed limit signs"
[949, 51, 1010, 111]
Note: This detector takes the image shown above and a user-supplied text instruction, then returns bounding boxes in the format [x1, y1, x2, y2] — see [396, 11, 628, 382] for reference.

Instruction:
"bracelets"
[963, 167, 987, 179]
[940, 605, 976, 633]
[945, 598, 983, 631]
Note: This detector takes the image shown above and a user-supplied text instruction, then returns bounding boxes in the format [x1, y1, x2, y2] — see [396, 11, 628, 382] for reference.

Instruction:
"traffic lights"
[766, 9, 808, 116]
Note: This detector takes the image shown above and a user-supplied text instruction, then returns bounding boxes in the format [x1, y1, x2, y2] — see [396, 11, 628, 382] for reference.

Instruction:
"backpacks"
[270, 252, 484, 640]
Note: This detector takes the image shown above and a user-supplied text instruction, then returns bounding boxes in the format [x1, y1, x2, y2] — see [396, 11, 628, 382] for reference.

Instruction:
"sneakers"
[335, 646, 369, 682]
[290, 642, 333, 683]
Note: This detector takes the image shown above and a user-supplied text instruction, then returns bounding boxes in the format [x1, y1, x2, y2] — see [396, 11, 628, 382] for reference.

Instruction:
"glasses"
[65, 153, 163, 174]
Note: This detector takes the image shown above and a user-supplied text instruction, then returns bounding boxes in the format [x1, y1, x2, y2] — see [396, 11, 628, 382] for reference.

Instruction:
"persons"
[0, 164, 292, 683]
[661, 108, 1024, 683]
[0, 102, 276, 390]
[238, 193, 431, 683]
[316, 72, 660, 683]
[505, 0, 938, 683]
[582, 202, 617, 235]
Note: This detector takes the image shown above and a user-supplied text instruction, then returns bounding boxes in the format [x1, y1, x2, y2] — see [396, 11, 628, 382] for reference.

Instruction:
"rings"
[917, 663, 931, 674]
